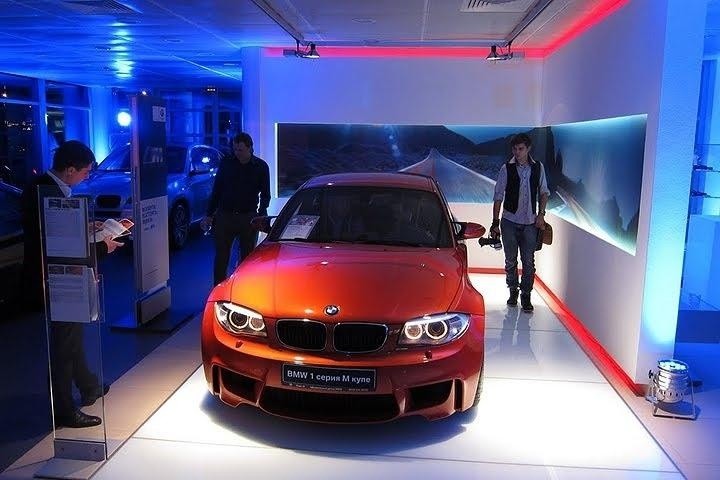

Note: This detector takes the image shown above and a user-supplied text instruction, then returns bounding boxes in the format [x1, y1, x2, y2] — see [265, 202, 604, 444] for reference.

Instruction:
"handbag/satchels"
[535, 221, 552, 252]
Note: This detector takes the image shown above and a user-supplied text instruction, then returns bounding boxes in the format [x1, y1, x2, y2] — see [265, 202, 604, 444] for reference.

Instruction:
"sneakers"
[506, 289, 519, 307]
[520, 292, 534, 312]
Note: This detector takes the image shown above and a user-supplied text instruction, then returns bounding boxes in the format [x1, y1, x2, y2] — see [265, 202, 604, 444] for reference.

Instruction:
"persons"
[488, 132, 552, 313]
[19, 140, 125, 430]
[200, 132, 271, 290]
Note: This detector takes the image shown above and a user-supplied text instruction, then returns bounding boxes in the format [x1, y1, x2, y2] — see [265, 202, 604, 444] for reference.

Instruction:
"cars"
[70, 142, 226, 256]
[200, 172, 486, 428]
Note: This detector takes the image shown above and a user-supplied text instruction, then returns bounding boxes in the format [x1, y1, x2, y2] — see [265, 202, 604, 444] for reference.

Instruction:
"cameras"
[477, 236, 502, 251]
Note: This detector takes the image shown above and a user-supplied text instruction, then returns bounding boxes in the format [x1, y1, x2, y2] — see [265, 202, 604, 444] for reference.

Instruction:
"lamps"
[485, 43, 513, 61]
[296, 40, 320, 59]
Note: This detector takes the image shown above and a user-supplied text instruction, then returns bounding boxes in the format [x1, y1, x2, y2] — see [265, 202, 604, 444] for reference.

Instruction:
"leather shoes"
[83, 384, 110, 406]
[51, 405, 102, 430]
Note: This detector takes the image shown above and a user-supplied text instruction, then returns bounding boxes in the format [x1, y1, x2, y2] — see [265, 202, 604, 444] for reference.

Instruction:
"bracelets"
[539, 209, 546, 215]
